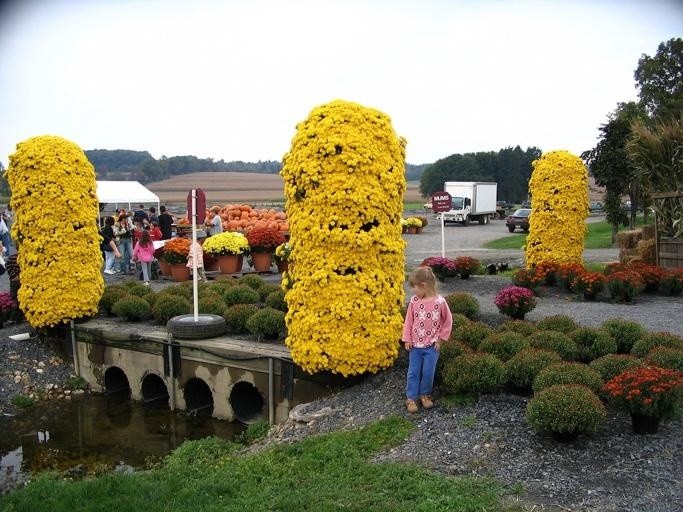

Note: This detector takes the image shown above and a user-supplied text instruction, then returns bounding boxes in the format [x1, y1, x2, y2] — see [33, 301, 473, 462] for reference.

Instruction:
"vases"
[628, 411, 659, 435]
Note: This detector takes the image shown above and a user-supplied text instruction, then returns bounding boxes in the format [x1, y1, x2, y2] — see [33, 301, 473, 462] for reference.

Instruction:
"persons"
[402, 266, 453, 413]
[1, 204, 24, 323]
[205, 207, 223, 237]
[97, 204, 173, 285]
[186, 235, 208, 284]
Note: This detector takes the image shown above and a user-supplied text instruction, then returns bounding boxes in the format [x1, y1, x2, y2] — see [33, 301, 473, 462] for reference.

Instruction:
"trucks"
[436, 180, 498, 227]
[495, 200, 510, 221]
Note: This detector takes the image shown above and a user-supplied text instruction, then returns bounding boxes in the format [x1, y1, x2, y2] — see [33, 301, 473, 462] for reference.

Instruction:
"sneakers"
[104, 268, 122, 275]
[144, 281, 150, 286]
[405, 399, 418, 413]
[420, 395, 434, 409]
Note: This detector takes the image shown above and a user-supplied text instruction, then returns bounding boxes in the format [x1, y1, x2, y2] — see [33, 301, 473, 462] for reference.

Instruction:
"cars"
[590, 201, 605, 210]
[623, 200, 632, 211]
[424, 200, 433, 209]
[520, 200, 532, 209]
[504, 208, 533, 233]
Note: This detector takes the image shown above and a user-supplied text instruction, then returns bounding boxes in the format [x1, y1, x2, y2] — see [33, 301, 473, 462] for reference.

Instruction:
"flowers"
[601, 363, 682, 426]
[419, 248, 681, 324]
[153, 221, 290, 278]
[399, 214, 427, 235]
